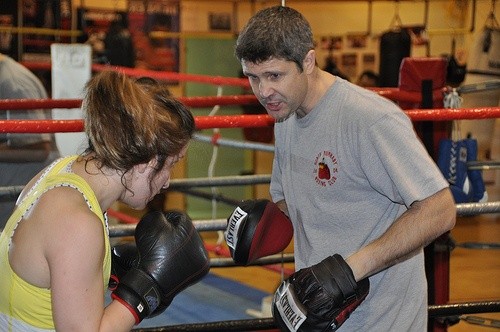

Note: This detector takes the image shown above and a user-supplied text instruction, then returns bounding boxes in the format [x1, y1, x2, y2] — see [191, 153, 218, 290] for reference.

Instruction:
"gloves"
[107, 243, 142, 291]
[223, 198, 293, 265]
[111, 205, 211, 325]
[273, 252, 370, 332]
[436, 138, 489, 204]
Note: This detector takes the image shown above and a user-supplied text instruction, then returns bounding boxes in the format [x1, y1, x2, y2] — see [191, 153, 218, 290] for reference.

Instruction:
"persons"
[0, 69, 210, 332]
[222, 6, 456, 332]
[0, 51, 58, 215]
[82, 12, 138, 69]
